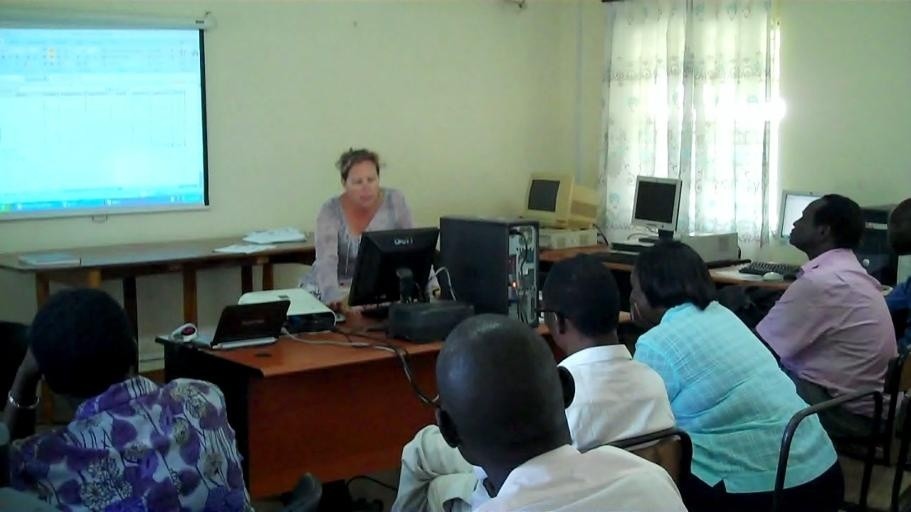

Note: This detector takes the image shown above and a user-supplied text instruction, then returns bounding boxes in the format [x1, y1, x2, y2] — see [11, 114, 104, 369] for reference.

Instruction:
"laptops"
[192, 300, 291, 351]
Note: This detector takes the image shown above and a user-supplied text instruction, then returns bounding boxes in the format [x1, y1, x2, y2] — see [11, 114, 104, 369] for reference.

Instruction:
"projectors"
[237, 288, 336, 337]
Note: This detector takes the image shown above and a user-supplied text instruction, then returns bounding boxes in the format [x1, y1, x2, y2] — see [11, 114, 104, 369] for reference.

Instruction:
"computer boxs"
[852, 201, 910, 290]
[538, 229, 598, 250]
[441, 217, 540, 321]
[611, 232, 738, 268]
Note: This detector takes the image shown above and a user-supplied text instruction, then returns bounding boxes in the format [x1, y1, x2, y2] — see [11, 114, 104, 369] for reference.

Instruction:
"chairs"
[768, 353, 910, 512]
[583, 426, 695, 507]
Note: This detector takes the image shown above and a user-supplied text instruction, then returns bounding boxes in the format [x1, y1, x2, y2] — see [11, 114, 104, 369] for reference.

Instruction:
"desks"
[156, 305, 632, 502]
[1, 234, 314, 340]
[538, 246, 794, 291]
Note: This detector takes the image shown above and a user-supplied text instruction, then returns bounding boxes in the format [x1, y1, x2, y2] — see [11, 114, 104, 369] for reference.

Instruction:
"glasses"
[532, 305, 572, 325]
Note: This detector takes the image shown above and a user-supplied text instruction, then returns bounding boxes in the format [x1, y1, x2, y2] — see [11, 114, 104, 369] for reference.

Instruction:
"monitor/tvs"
[631, 175, 683, 243]
[775, 189, 828, 241]
[528, 172, 599, 229]
[348, 226, 440, 310]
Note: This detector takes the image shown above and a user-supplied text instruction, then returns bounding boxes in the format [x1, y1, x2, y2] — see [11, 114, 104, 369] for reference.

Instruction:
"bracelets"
[7, 389, 40, 410]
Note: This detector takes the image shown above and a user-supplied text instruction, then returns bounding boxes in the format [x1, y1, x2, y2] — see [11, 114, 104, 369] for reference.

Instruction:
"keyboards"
[739, 261, 801, 277]
[589, 251, 639, 264]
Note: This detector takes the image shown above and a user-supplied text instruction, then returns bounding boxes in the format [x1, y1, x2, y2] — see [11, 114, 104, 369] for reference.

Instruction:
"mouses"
[763, 272, 784, 281]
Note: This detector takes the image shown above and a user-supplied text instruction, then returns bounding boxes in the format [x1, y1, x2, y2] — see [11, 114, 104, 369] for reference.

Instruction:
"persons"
[880, 199, 910, 350]
[299, 149, 441, 319]
[751, 193, 903, 450]
[627, 238, 845, 511]
[390, 255, 679, 511]
[0, 285, 254, 512]
[435, 313, 686, 510]
